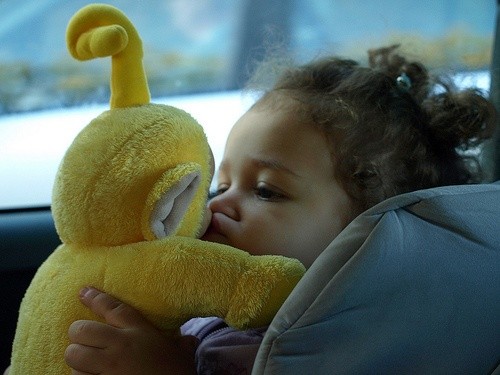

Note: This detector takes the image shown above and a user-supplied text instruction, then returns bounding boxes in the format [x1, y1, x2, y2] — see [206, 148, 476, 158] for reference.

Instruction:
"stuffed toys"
[6, 3, 306, 374]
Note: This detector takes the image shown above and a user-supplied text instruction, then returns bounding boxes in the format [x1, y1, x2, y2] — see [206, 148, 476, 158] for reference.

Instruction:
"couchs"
[250, 177, 500, 375]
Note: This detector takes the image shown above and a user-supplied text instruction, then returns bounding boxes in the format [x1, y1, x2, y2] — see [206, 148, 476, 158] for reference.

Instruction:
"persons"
[64, 42, 499, 374]
